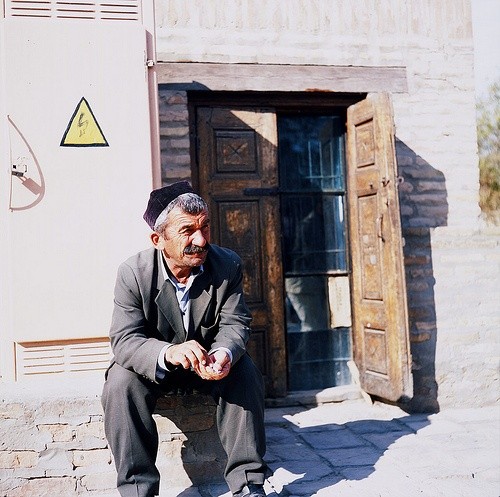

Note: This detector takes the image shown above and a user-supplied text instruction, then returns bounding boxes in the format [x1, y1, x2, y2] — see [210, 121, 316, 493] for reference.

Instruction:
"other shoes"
[233, 484, 267, 497]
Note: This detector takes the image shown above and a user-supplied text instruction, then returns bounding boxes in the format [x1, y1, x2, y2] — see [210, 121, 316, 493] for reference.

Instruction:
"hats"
[143, 181, 196, 231]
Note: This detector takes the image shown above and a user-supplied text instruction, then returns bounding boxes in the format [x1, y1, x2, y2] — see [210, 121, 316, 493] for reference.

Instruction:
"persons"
[101, 182, 268, 497]
